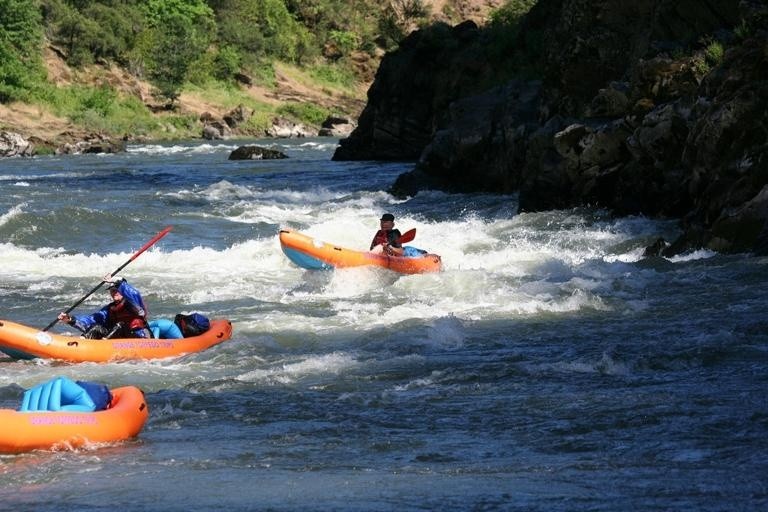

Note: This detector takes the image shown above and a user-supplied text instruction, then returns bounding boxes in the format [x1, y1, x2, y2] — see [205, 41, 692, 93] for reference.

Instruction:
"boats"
[278, 229, 442, 274]
[0, 318, 233, 363]
[0, 385, 150, 452]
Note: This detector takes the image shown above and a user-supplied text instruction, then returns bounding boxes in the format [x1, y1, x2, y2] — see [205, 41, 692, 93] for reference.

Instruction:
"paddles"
[386, 229, 415, 246]
[42, 226, 173, 332]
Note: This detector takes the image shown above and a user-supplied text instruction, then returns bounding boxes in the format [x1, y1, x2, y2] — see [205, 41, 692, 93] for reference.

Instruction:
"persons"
[372, 214, 403, 257]
[57, 274, 152, 339]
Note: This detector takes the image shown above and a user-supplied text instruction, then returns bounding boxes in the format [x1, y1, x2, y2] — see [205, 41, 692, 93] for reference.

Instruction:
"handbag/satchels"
[173, 314, 209, 336]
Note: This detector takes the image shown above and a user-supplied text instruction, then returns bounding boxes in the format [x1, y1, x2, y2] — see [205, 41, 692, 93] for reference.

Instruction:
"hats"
[380, 214, 395, 221]
[105, 277, 127, 289]
